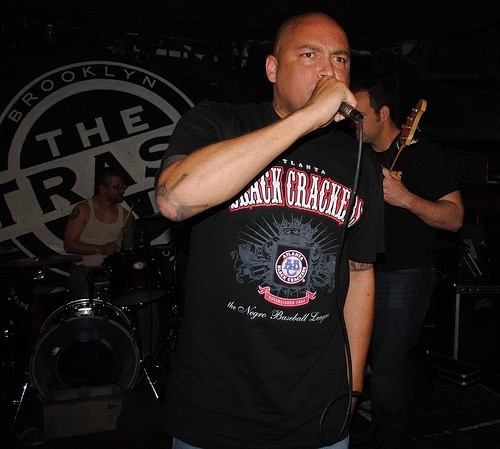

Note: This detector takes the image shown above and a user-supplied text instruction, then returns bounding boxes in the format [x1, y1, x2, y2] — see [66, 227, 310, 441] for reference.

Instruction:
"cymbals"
[12, 255, 84, 270]
[122, 213, 169, 247]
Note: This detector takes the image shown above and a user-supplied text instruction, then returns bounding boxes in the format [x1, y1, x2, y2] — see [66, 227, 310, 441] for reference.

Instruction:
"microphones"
[337, 102, 364, 122]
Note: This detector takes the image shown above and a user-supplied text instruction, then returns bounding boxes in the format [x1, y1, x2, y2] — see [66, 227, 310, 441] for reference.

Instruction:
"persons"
[153, 10, 382, 449]
[346, 67, 464, 449]
[63, 166, 135, 283]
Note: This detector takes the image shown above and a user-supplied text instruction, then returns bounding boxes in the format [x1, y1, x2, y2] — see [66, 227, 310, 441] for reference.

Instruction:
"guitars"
[386, 99, 427, 170]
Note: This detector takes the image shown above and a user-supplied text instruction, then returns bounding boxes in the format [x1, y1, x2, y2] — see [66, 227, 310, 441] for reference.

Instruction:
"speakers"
[435, 278, 500, 365]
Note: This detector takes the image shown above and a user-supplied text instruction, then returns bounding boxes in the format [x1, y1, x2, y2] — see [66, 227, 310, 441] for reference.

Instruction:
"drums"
[28, 296, 142, 399]
[99, 245, 183, 307]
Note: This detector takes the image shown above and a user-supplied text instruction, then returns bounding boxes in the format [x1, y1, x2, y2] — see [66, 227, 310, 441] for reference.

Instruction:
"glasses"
[105, 184, 127, 190]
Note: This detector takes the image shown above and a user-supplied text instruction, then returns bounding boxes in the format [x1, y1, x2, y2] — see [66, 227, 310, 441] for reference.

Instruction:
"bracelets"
[352, 390, 361, 399]
